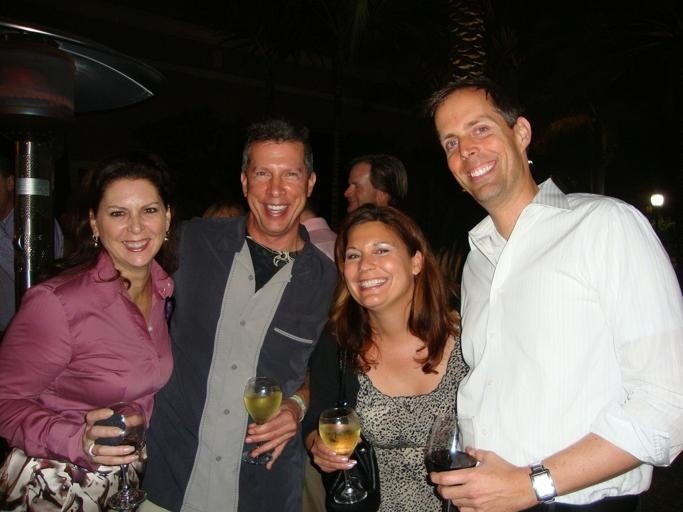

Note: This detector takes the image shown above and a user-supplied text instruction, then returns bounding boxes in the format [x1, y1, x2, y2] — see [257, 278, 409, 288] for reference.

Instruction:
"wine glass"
[96, 401, 148, 511]
[316, 406, 369, 505]
[423, 416, 477, 512]
[239, 376, 282, 467]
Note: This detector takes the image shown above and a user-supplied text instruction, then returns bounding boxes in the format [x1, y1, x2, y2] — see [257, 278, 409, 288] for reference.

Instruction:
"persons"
[2, 77, 683, 511]
